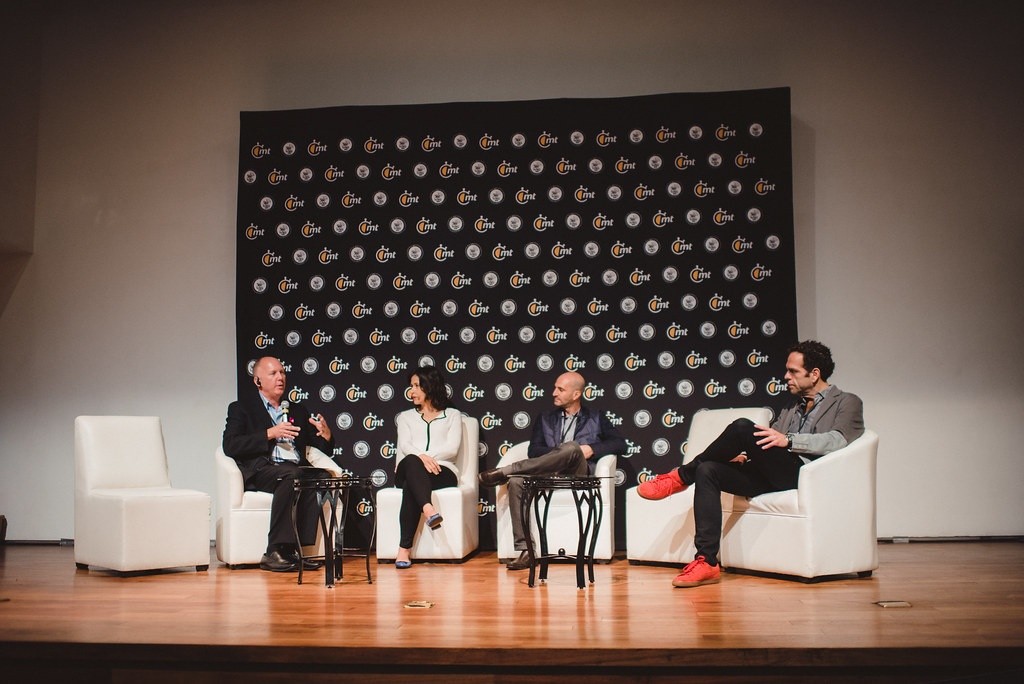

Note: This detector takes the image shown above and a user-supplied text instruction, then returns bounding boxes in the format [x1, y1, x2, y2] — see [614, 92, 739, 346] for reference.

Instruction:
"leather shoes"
[260, 551, 296, 571]
[477, 467, 509, 487]
[506, 550, 539, 570]
[282, 550, 322, 569]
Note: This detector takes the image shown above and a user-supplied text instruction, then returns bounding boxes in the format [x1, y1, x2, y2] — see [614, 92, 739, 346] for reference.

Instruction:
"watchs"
[785, 433, 793, 449]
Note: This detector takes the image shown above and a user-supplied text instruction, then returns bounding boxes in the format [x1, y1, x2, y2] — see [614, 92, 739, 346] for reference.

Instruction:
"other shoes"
[395, 557, 412, 568]
[426, 513, 443, 531]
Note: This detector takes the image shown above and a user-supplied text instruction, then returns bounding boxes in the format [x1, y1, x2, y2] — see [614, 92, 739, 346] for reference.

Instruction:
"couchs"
[494, 441, 616, 565]
[215, 444, 343, 569]
[376, 416, 480, 564]
[625, 408, 880, 584]
[73, 415, 210, 579]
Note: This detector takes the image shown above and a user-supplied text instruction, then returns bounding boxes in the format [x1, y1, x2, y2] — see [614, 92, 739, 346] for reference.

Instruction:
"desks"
[276, 476, 386, 589]
[507, 474, 614, 590]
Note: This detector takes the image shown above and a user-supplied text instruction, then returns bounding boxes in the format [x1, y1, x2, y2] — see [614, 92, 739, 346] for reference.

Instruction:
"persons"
[634, 340, 865, 588]
[221, 355, 337, 572]
[476, 372, 627, 571]
[394, 365, 464, 568]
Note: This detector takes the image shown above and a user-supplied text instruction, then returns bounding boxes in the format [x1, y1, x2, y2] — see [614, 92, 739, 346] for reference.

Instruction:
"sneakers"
[637, 468, 688, 501]
[672, 555, 720, 586]
